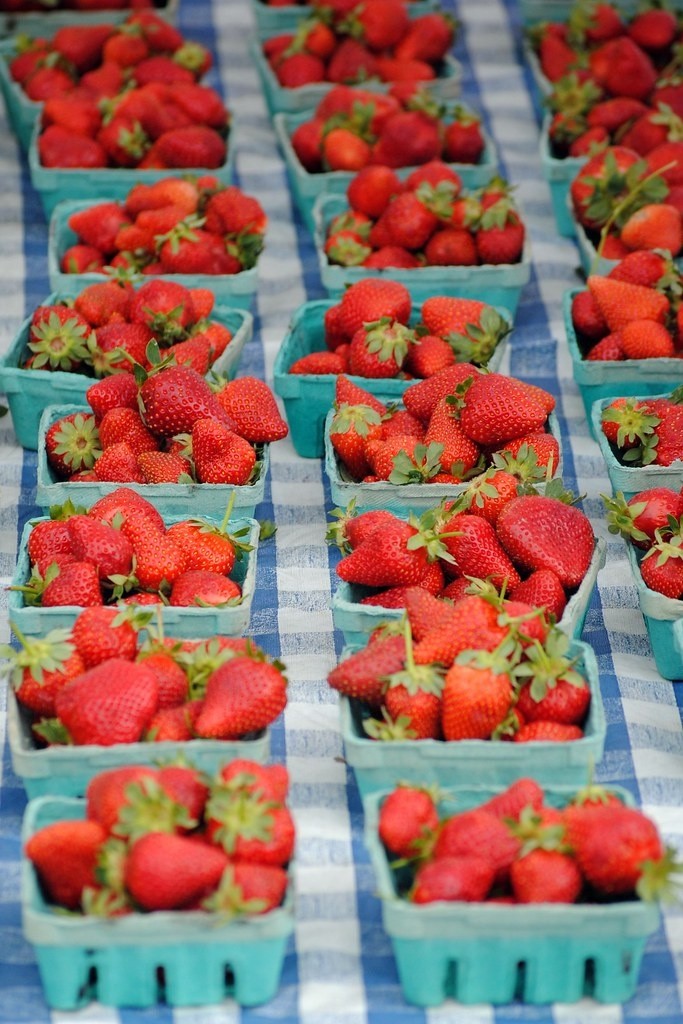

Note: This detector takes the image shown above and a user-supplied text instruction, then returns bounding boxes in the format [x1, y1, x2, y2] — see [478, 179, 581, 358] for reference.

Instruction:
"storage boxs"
[0, 9, 289, 1009]
[249, 0, 682, 1007]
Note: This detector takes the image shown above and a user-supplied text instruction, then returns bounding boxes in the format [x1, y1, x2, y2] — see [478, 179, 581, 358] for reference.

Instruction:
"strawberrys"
[1, 0, 683, 917]
